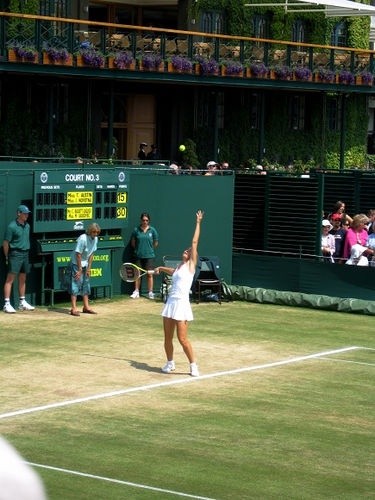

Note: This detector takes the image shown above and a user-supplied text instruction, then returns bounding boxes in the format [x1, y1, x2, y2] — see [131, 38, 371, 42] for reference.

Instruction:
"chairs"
[161, 255, 183, 304]
[192, 256, 222, 304]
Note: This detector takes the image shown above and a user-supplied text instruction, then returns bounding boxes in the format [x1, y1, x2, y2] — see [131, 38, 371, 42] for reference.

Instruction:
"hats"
[332, 213, 342, 221]
[151, 144, 157, 148]
[17, 205, 31, 214]
[140, 142, 147, 145]
[351, 244, 368, 258]
[255, 165, 263, 170]
[206, 161, 218, 168]
[322, 219, 333, 231]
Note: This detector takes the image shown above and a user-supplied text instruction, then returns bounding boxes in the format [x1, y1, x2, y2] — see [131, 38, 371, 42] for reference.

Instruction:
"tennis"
[180, 145, 186, 151]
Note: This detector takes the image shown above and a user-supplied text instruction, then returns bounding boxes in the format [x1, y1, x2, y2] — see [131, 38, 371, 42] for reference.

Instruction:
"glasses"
[142, 220, 149, 222]
[212, 165, 218, 167]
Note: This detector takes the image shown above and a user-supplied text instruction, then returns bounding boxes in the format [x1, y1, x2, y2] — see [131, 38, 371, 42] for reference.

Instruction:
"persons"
[168, 164, 201, 176]
[202, 161, 218, 175]
[129, 213, 159, 299]
[344, 214, 375, 259]
[137, 142, 148, 165]
[341, 219, 351, 231]
[2, 204, 35, 313]
[255, 164, 264, 175]
[328, 213, 348, 263]
[345, 244, 369, 266]
[365, 222, 375, 260]
[154, 210, 205, 377]
[218, 160, 233, 176]
[321, 220, 336, 264]
[328, 201, 354, 223]
[67, 223, 101, 316]
[367, 209, 375, 235]
[147, 144, 161, 157]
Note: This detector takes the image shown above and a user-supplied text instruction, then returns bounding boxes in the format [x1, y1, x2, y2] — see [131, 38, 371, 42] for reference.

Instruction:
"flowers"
[44, 47, 69, 62]
[361, 71, 372, 83]
[171, 55, 191, 71]
[274, 65, 289, 79]
[81, 48, 102, 67]
[192, 54, 217, 75]
[338, 70, 355, 83]
[114, 51, 131, 69]
[295, 67, 311, 79]
[143, 53, 162, 69]
[13, 45, 36, 59]
[318, 68, 333, 81]
[249, 62, 266, 77]
[224, 61, 242, 73]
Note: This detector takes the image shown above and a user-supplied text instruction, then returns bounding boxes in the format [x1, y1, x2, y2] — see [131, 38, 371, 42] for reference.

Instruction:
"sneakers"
[83, 308, 96, 314]
[3, 304, 17, 313]
[71, 309, 80, 316]
[149, 292, 155, 300]
[130, 290, 139, 298]
[162, 362, 175, 372]
[190, 365, 199, 376]
[19, 301, 35, 312]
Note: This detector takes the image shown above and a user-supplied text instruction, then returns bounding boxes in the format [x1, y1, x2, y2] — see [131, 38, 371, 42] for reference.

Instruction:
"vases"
[42, 51, 73, 66]
[195, 63, 219, 76]
[109, 56, 136, 70]
[77, 52, 105, 69]
[168, 60, 192, 73]
[335, 74, 356, 84]
[270, 69, 291, 80]
[292, 70, 312, 82]
[7, 48, 39, 64]
[222, 65, 244, 78]
[356, 76, 374, 86]
[139, 58, 165, 72]
[246, 68, 268, 79]
[315, 71, 335, 84]
